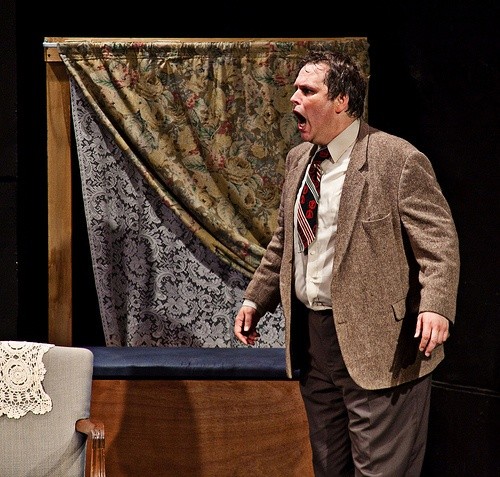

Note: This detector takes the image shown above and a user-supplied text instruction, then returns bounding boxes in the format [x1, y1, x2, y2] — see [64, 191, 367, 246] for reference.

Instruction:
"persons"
[232, 50, 461, 477]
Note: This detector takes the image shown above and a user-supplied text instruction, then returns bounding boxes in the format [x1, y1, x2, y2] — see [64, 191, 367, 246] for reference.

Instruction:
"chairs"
[0, 340, 106, 477]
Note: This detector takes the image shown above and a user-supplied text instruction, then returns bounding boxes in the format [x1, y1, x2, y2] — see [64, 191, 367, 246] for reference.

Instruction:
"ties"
[296, 147, 330, 253]
